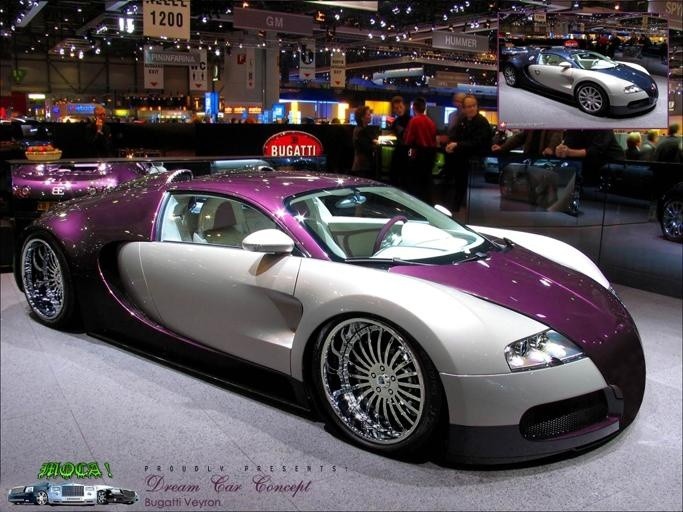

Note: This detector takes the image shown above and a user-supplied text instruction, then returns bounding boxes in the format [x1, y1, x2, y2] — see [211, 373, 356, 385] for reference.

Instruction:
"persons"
[80, 104, 113, 158]
[490, 120, 522, 157]
[403, 95, 439, 205]
[542, 128, 627, 216]
[386, 95, 413, 189]
[492, 127, 563, 214]
[446, 91, 466, 130]
[563, 30, 667, 65]
[443, 93, 494, 215]
[639, 131, 661, 161]
[653, 121, 683, 162]
[349, 105, 380, 179]
[621, 131, 643, 160]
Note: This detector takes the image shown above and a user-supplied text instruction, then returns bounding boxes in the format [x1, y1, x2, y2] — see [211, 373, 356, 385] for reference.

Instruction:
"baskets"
[25, 148, 62, 161]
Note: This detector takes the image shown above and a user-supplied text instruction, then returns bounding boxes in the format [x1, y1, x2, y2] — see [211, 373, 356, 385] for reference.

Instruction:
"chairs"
[162, 195, 312, 250]
[536, 159, 656, 206]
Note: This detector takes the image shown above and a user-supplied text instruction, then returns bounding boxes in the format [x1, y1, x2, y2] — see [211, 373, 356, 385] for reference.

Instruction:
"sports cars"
[502, 42, 668, 118]
[12, 160, 646, 469]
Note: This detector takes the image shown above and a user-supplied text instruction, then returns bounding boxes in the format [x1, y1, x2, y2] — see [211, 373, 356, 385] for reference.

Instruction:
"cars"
[483, 143, 683, 244]
